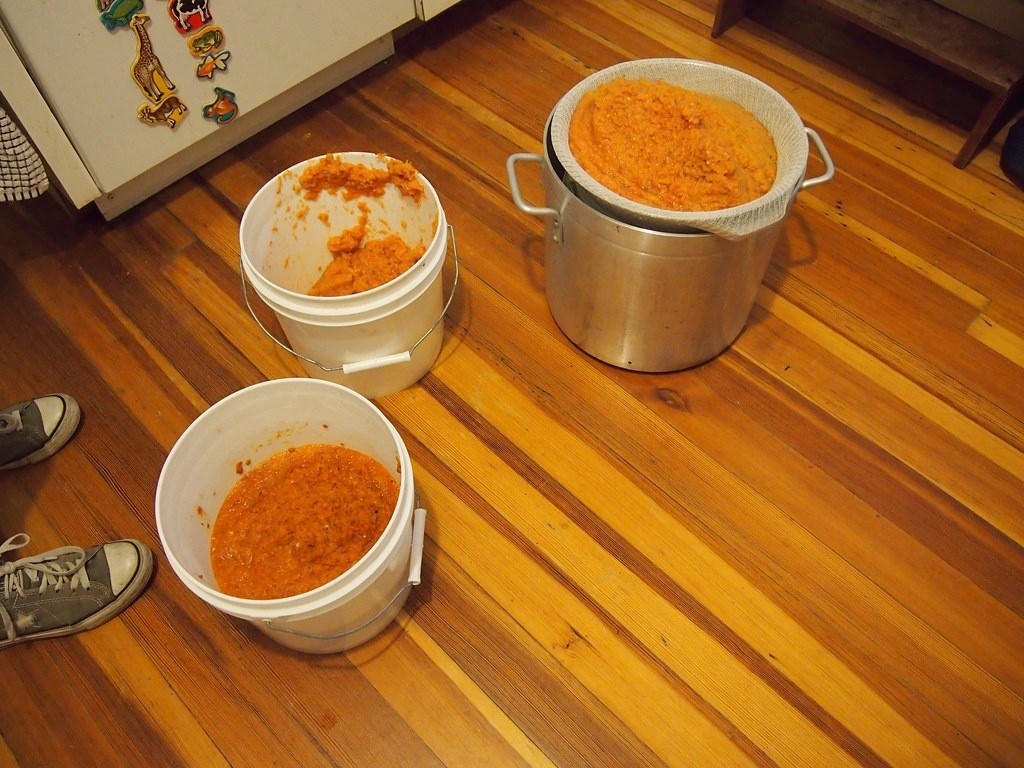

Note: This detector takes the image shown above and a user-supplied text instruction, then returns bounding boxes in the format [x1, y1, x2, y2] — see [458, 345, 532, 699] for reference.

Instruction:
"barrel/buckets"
[155, 378, 426, 657]
[235, 152, 460, 402]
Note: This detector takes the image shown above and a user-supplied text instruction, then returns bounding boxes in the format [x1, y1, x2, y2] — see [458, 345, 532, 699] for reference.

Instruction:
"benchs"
[711, 0, 1024, 171]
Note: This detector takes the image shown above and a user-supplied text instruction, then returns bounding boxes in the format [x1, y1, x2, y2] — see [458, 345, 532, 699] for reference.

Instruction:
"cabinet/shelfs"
[1, 0, 463, 223]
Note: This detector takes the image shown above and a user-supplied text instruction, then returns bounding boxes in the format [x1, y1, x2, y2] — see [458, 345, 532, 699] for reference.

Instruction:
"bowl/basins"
[552, 56, 808, 240]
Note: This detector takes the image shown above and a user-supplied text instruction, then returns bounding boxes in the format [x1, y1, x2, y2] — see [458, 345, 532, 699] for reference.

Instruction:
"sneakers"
[0, 393, 81, 472]
[0, 539, 153, 649]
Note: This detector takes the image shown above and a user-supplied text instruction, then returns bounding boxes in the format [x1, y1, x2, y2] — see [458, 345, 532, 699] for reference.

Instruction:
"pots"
[506, 57, 833, 375]
